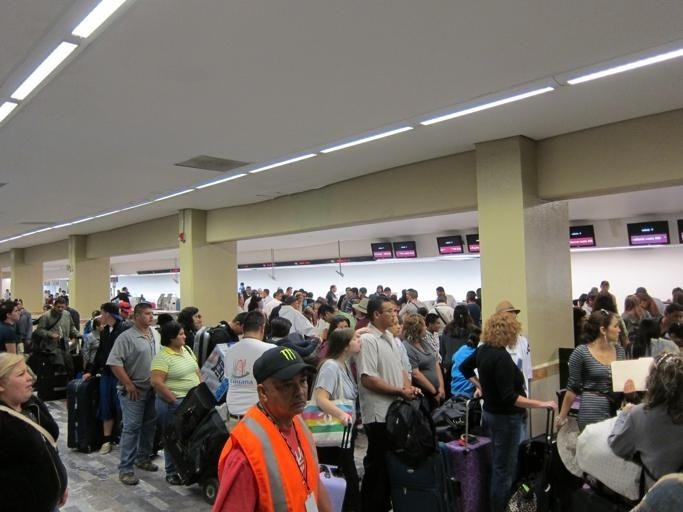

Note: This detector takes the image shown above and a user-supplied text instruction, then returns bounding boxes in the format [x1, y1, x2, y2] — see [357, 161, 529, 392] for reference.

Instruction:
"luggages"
[24, 335, 84, 401]
[158, 381, 230, 486]
[385, 393, 573, 510]
[65, 372, 122, 453]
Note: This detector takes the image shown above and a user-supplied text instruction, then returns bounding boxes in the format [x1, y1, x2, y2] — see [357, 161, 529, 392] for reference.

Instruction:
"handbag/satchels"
[301, 398, 356, 449]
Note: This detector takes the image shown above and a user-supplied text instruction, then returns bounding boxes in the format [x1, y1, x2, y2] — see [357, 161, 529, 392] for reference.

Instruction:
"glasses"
[655, 353, 671, 369]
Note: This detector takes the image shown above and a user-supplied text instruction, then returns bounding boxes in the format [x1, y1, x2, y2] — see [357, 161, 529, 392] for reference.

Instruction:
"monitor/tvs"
[677, 219, 683, 244]
[394, 241, 416, 258]
[437, 236, 463, 254]
[570, 225, 596, 247]
[466, 234, 480, 253]
[627, 221, 670, 245]
[371, 243, 393, 259]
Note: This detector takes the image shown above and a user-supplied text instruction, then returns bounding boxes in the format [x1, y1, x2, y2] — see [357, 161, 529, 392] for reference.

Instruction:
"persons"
[0, 282, 683, 512]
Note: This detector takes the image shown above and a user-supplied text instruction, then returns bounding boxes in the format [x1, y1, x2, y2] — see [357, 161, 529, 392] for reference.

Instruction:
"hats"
[284, 292, 302, 305]
[489, 301, 520, 317]
[101, 303, 122, 321]
[252, 346, 318, 384]
[351, 298, 369, 314]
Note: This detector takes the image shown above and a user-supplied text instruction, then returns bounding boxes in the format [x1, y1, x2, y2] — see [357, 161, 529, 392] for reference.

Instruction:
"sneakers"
[98, 440, 181, 485]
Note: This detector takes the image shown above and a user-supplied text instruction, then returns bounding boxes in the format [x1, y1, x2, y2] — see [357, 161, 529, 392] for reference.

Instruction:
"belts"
[226, 407, 244, 420]
